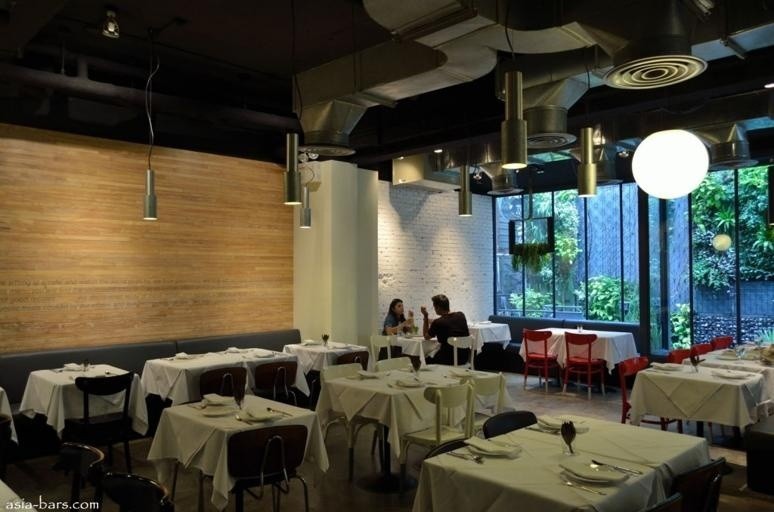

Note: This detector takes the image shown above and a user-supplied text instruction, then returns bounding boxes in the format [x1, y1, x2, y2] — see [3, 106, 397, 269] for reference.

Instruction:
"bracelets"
[423, 318, 429, 321]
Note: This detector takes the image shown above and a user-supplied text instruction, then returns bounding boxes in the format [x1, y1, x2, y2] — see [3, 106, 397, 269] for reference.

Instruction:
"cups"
[577, 324, 583, 332]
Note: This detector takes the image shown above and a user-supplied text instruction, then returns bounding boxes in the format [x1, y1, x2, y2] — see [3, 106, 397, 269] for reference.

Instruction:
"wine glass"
[735, 347, 745, 364]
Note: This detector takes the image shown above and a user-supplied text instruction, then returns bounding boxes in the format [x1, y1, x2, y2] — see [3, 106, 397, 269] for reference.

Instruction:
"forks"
[559, 475, 606, 496]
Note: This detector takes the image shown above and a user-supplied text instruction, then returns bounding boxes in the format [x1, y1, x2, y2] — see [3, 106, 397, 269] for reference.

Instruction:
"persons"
[421, 294, 470, 366]
[379, 299, 414, 360]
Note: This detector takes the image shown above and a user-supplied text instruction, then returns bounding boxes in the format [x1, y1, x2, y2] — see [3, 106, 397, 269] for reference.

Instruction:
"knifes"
[591, 459, 643, 475]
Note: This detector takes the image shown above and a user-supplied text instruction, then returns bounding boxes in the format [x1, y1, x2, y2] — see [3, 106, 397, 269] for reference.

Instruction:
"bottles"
[397, 321, 403, 338]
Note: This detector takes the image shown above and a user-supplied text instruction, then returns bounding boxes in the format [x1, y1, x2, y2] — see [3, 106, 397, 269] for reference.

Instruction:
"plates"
[562, 462, 629, 485]
[539, 416, 592, 432]
[450, 372, 477, 378]
[718, 372, 751, 379]
[468, 439, 522, 457]
[335, 346, 352, 349]
[655, 363, 684, 371]
[176, 355, 191, 359]
[238, 409, 276, 421]
[400, 379, 424, 387]
[203, 406, 236, 416]
[256, 352, 274, 358]
[202, 397, 235, 405]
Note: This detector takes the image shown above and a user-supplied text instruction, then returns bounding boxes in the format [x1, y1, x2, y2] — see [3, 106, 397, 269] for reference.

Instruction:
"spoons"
[589, 463, 633, 476]
[526, 426, 560, 434]
[105, 369, 120, 376]
[445, 450, 484, 462]
[234, 414, 253, 427]
